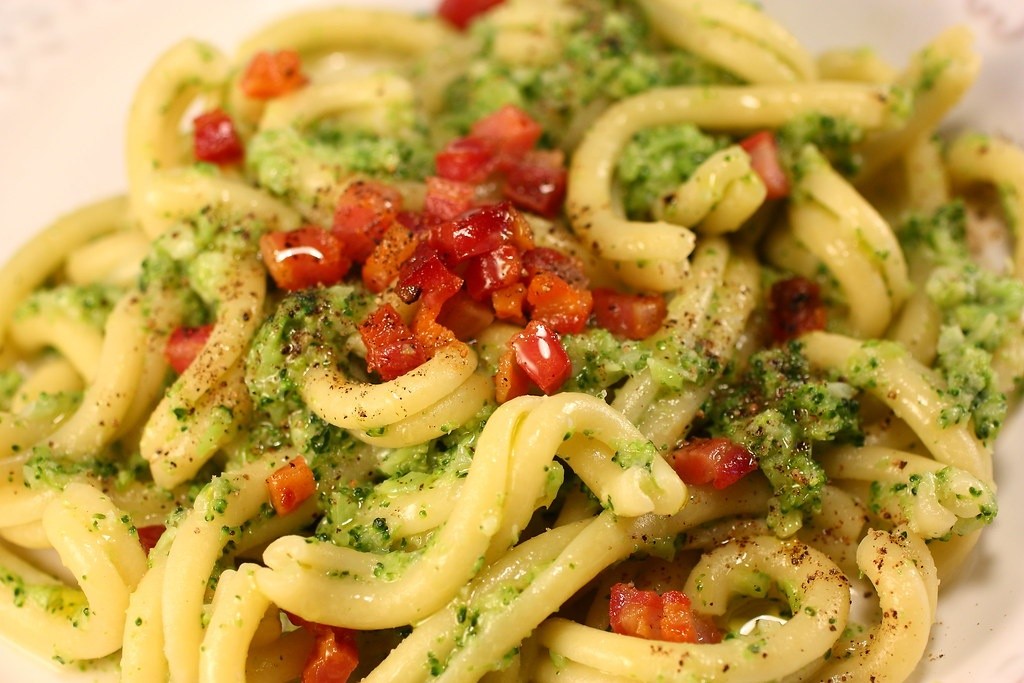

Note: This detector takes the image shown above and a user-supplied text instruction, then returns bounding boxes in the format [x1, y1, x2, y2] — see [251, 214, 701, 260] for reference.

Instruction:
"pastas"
[0, 1, 1024, 683]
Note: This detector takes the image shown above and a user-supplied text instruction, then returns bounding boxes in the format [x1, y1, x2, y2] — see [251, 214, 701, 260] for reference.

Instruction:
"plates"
[1, 2, 1023, 681]
